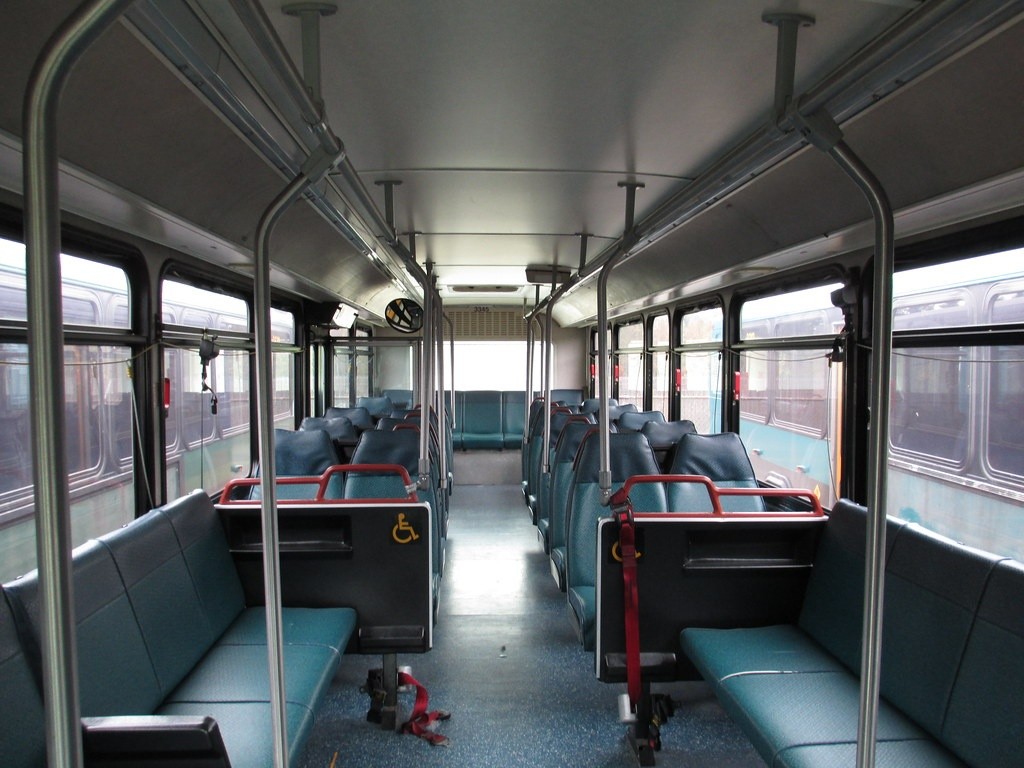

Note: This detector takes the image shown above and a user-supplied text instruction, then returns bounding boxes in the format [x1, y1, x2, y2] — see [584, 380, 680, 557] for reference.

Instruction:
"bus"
[1, 237, 336, 590]
[622, 338, 735, 430]
[707, 243, 1023, 580]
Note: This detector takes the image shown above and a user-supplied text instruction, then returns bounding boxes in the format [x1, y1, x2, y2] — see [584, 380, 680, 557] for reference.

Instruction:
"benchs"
[679, 496, 1024, 768]
[0, 487, 358, 768]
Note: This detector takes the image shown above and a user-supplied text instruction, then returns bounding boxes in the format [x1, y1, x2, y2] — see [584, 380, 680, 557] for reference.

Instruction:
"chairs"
[252, 383, 765, 627]
